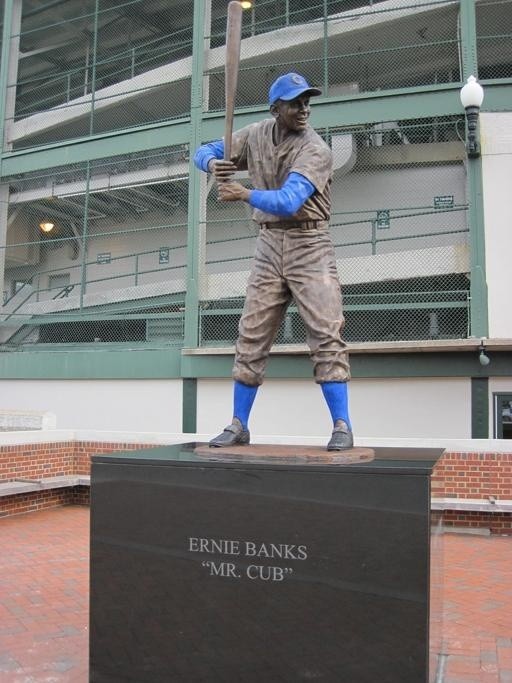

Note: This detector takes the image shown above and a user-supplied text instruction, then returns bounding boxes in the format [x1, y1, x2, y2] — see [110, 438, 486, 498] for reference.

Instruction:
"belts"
[259, 219, 329, 230]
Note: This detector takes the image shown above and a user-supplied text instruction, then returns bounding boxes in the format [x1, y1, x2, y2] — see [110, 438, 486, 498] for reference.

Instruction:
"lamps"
[458, 72, 486, 160]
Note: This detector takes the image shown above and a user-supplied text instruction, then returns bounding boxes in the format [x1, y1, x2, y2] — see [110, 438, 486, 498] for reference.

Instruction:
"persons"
[192, 71, 355, 453]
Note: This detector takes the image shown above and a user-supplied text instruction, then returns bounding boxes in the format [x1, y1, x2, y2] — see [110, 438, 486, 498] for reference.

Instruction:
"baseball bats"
[219, 2, 242, 183]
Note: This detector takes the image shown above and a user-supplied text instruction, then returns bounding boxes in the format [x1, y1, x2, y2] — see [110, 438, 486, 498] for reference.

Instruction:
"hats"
[269, 72, 322, 109]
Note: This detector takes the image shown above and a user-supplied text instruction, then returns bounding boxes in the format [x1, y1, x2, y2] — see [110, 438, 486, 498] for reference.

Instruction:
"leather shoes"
[326, 425, 354, 451]
[208, 417, 250, 448]
